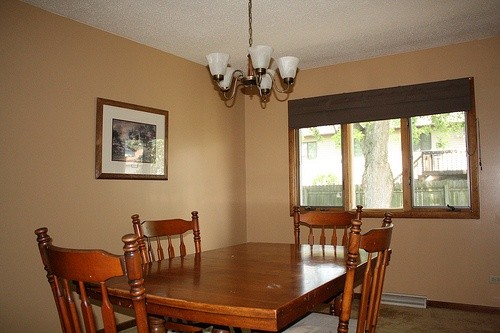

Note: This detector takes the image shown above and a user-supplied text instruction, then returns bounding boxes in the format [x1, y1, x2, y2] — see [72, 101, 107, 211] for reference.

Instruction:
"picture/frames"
[95, 97, 168, 180]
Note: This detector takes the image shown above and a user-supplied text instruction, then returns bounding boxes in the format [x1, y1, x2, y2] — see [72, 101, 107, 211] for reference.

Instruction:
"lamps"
[206, 0, 300, 102]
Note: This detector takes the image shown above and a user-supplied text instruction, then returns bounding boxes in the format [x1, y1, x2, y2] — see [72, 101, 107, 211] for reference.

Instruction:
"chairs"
[251, 212, 394, 333]
[35, 227, 149, 333]
[294, 205, 363, 317]
[131, 211, 242, 333]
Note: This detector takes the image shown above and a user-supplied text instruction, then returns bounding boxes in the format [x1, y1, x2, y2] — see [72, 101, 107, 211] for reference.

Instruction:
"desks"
[72, 242, 392, 333]
